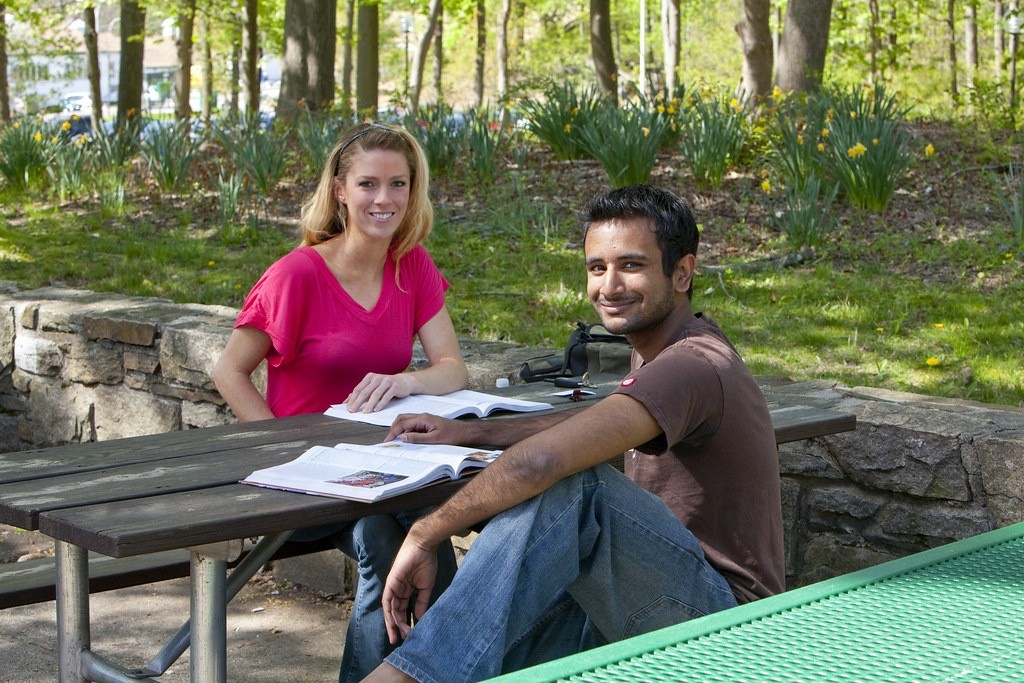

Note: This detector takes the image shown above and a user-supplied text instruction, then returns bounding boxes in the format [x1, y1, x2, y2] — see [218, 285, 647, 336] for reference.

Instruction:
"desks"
[0, 372, 858, 683]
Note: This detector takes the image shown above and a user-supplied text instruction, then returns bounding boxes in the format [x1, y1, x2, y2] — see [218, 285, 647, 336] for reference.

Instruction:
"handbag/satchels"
[519, 354, 565, 382]
[565, 321, 633, 376]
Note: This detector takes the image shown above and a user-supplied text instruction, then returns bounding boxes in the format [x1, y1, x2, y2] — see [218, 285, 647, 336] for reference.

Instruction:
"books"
[239, 390, 553, 503]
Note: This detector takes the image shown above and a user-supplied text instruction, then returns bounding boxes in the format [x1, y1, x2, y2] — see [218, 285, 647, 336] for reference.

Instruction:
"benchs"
[0, 535, 345, 609]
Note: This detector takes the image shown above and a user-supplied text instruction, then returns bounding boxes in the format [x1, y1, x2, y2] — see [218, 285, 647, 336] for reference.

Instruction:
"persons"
[211, 121, 469, 683]
[359, 186, 786, 683]
